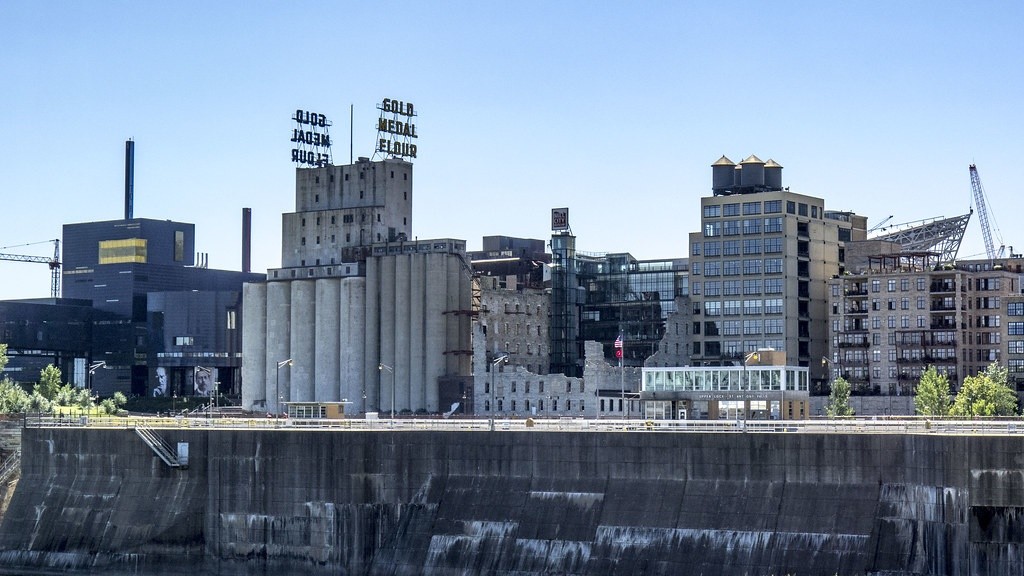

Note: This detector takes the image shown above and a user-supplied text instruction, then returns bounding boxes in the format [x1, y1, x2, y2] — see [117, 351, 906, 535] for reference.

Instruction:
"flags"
[614, 334, 622, 347]
[615, 349, 623, 358]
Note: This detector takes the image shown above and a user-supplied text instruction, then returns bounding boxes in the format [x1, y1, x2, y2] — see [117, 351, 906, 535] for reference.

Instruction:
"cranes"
[970, 164, 1004, 258]
[0, 238, 62, 295]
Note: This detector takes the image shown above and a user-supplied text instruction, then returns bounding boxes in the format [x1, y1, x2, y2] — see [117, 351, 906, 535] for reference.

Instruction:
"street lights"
[822, 356, 837, 421]
[379, 361, 396, 419]
[196, 364, 214, 421]
[489, 352, 509, 431]
[275, 358, 293, 419]
[86, 360, 106, 419]
[743, 350, 758, 432]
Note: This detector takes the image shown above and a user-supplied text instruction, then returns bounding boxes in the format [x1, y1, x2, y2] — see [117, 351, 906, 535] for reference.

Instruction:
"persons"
[151, 367, 170, 397]
[180, 368, 193, 395]
[194, 370, 214, 397]
